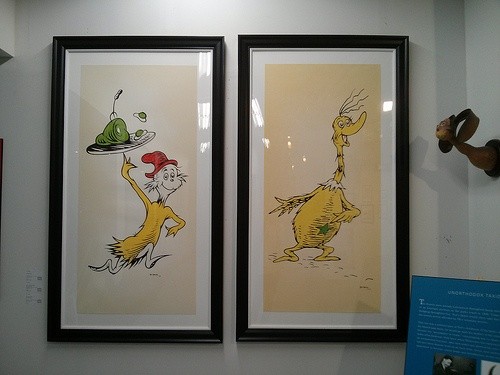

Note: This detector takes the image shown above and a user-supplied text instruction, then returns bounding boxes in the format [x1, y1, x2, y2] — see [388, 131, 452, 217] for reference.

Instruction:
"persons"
[434, 354, 455, 375]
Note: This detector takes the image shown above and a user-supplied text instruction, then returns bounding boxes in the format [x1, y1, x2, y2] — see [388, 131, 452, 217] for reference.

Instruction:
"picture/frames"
[44, 34, 225, 346]
[234, 33, 411, 345]
